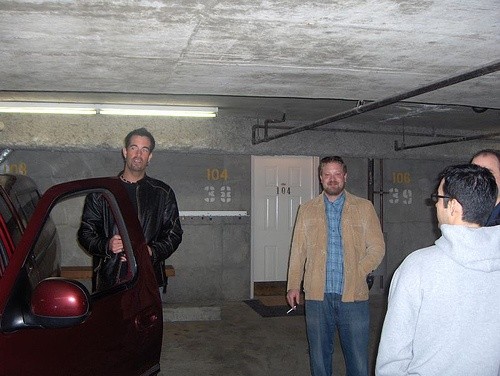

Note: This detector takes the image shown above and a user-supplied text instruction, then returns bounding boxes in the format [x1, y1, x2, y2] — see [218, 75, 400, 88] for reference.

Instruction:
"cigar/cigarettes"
[287, 305, 296, 314]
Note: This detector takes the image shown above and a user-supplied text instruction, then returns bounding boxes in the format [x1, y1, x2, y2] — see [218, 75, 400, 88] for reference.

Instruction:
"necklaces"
[121, 175, 132, 184]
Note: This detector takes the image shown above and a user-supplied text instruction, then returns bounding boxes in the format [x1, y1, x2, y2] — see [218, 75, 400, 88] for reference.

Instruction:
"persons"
[374, 163, 500, 376]
[77, 128, 183, 294]
[286, 155, 386, 376]
[470, 150, 500, 226]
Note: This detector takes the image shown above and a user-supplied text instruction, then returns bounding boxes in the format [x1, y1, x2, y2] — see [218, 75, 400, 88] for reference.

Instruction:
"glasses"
[431, 194, 456, 204]
[129, 146, 149, 154]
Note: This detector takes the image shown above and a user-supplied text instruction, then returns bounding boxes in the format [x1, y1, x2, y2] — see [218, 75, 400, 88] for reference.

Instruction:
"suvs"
[0, 173, 163, 376]
